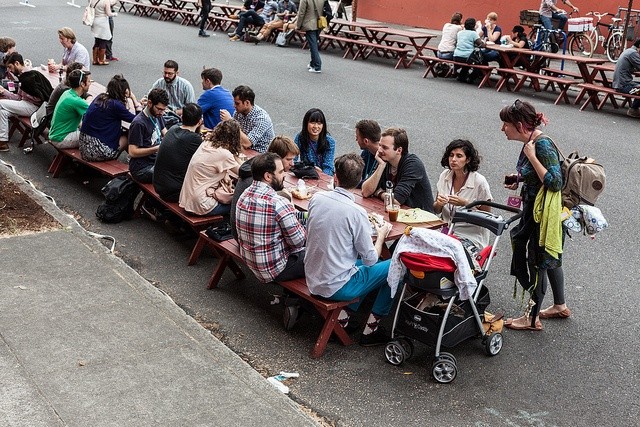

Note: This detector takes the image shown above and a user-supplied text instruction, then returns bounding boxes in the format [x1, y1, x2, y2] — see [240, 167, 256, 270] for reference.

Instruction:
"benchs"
[538, 65, 614, 91]
[419, 56, 496, 89]
[200, 227, 360, 360]
[498, 68, 581, 107]
[127, 170, 224, 235]
[577, 83, 639, 111]
[378, 37, 439, 59]
[237, 21, 283, 43]
[303, 33, 355, 58]
[48, 139, 132, 177]
[5, 96, 51, 149]
[352, 41, 413, 69]
[326, 29, 370, 49]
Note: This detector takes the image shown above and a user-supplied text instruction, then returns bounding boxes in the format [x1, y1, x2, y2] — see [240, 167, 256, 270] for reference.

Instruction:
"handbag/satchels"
[205, 177, 236, 204]
[28, 101, 52, 139]
[313, 0, 327, 28]
[288, 162, 320, 179]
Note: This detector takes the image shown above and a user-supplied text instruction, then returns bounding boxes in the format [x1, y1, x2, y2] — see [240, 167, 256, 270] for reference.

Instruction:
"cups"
[386, 204, 400, 222]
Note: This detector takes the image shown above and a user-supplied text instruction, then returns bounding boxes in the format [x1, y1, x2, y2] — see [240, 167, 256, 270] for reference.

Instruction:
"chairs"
[108, 0, 318, 38]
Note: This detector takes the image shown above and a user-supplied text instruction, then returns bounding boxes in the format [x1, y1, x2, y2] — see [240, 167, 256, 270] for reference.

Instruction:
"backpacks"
[519, 135, 607, 236]
[96, 175, 138, 223]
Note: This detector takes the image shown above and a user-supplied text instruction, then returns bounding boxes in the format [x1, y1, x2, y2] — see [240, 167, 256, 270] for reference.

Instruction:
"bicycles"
[574, 11, 629, 64]
[522, 23, 568, 76]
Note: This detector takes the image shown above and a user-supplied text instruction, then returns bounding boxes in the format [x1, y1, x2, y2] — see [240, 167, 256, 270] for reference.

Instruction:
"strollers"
[518, 7, 593, 60]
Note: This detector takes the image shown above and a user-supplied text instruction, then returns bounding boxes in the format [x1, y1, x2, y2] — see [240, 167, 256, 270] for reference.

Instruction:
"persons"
[179, 120, 243, 216]
[476, 12, 501, 61]
[231, 136, 300, 244]
[88, 0, 118, 65]
[612, 39, 640, 118]
[355, 119, 382, 188]
[176, 69, 236, 128]
[129, 89, 170, 222]
[235, 153, 307, 332]
[362, 128, 434, 214]
[0, 38, 16, 80]
[436, 13, 464, 60]
[499, 99, 571, 331]
[453, 18, 485, 82]
[220, 85, 275, 152]
[297, 0, 329, 72]
[506, 26, 531, 65]
[0, 52, 53, 151]
[106, 1, 119, 61]
[48, 27, 92, 74]
[227, 0, 298, 47]
[292, 108, 335, 176]
[434, 139, 492, 250]
[199, 0, 213, 37]
[141, 60, 195, 115]
[153, 102, 204, 236]
[539, 0, 579, 42]
[48, 70, 91, 149]
[79, 74, 141, 162]
[303, 154, 399, 346]
[44, 63, 93, 129]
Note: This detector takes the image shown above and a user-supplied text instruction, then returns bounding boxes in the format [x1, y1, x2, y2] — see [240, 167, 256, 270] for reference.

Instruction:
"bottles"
[385, 180, 393, 210]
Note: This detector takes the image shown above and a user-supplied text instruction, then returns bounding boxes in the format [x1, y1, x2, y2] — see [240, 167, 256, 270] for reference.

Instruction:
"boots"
[98, 49, 109, 65]
[93, 47, 99, 65]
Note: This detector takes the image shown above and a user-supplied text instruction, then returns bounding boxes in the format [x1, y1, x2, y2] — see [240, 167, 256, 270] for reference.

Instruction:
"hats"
[68, 69, 91, 86]
[511, 26, 524, 33]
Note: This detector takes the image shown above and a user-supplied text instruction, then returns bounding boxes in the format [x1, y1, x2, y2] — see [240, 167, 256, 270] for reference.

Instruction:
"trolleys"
[383, 200, 525, 387]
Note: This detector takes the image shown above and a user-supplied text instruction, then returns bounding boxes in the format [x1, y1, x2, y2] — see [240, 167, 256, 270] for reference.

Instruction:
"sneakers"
[626, 110, 640, 119]
[308, 64, 314, 69]
[0, 143, 10, 152]
[360, 325, 390, 347]
[309, 68, 321, 73]
[282, 304, 300, 331]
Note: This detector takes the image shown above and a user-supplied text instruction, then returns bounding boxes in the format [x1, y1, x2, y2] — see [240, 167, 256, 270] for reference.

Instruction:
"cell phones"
[506, 173, 520, 188]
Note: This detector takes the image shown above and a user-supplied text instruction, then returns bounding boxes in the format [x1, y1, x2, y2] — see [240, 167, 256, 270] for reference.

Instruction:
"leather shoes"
[503, 317, 543, 331]
[539, 308, 570, 318]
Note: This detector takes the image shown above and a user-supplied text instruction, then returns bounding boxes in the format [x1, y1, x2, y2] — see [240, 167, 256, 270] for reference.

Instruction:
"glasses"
[511, 98, 527, 119]
[162, 71, 175, 75]
